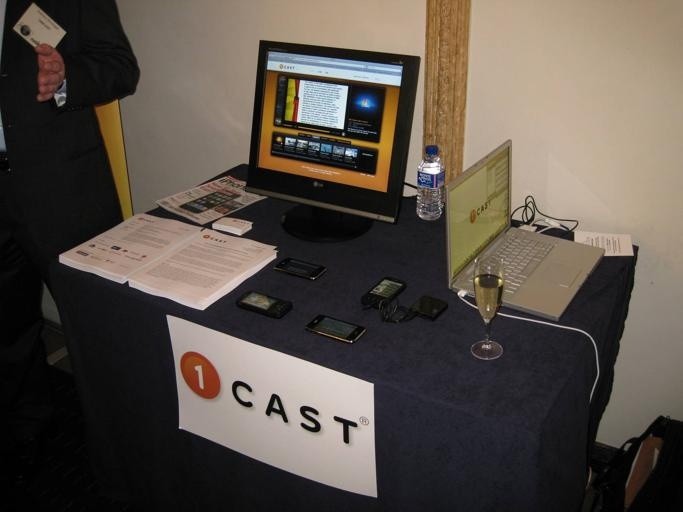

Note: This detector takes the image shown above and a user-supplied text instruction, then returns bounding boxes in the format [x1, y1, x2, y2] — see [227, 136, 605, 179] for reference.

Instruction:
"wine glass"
[470, 255, 507, 362]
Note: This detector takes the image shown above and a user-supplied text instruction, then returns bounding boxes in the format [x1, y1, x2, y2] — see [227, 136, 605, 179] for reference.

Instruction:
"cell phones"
[307, 314, 366, 344]
[274, 256, 327, 279]
[361, 275, 406, 311]
[239, 291, 292, 317]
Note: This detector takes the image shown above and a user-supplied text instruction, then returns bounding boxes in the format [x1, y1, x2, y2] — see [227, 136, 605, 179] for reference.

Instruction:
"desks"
[48, 163, 639, 507]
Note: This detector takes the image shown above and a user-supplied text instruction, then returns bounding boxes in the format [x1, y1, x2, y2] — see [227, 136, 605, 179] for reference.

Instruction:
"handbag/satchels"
[599, 416, 683, 510]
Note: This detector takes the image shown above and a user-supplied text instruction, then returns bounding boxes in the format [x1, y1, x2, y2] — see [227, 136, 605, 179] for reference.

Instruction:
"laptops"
[447, 139, 604, 320]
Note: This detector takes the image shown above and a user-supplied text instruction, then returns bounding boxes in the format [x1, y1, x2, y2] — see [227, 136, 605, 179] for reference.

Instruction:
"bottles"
[416, 146, 446, 221]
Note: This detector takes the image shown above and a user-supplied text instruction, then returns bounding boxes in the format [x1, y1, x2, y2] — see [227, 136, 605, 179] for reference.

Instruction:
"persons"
[0, 1, 142, 431]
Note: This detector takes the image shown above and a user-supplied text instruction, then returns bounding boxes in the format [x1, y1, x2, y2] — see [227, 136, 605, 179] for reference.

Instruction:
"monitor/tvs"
[246, 38, 421, 244]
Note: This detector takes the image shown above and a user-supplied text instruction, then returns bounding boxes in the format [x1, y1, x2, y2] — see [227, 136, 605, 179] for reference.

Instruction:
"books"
[58, 212, 279, 311]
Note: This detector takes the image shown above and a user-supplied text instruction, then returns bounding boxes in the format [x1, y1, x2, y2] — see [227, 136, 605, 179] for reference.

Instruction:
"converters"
[415, 296, 448, 320]
[544, 220, 560, 227]
